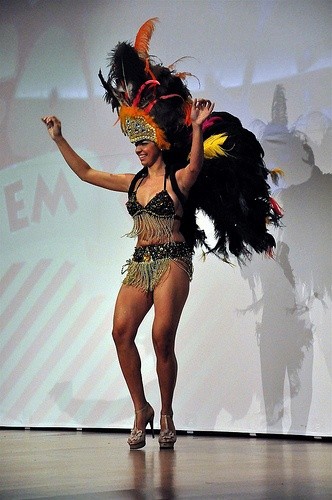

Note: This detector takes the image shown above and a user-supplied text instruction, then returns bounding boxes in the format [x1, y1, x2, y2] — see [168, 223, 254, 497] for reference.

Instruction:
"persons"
[41, 98, 215, 450]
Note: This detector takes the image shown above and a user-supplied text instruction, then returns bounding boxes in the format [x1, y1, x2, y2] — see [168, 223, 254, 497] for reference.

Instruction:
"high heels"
[159, 410, 176, 447]
[127, 402, 155, 449]
